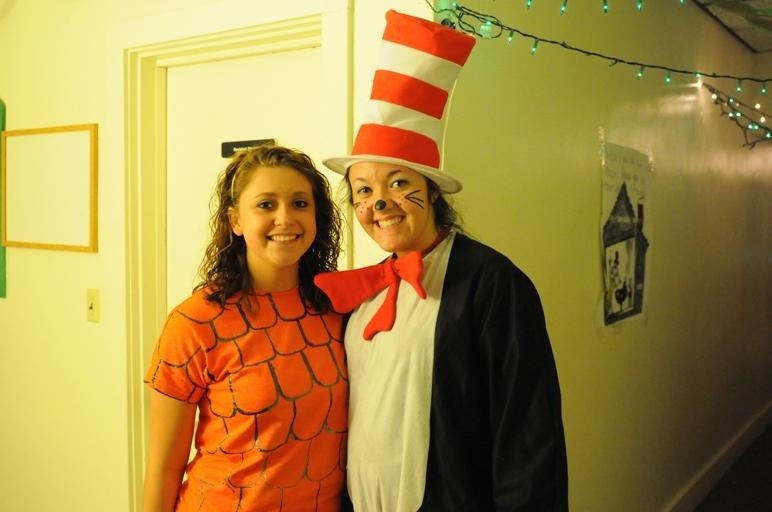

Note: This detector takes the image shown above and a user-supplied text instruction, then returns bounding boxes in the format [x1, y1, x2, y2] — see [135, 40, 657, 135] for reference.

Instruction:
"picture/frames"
[1, 124, 98, 253]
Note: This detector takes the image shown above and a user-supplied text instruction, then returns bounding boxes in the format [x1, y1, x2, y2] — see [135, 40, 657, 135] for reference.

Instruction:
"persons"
[138, 144, 347, 512]
[325, 157, 567, 509]
[610, 250, 621, 304]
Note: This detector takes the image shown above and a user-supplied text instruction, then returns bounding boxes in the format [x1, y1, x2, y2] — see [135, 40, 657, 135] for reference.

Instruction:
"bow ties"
[314, 250, 428, 341]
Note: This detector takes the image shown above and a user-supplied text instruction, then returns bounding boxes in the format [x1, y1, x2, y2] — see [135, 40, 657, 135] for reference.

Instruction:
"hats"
[322, 9, 477, 194]
